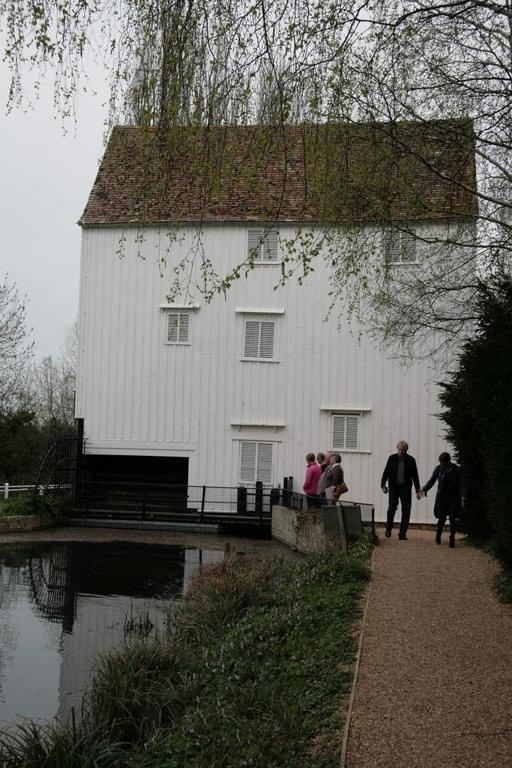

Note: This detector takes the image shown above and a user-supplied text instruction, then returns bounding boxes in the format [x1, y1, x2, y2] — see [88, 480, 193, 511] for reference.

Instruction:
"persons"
[327, 454, 343, 505]
[380, 441, 422, 539]
[303, 453, 322, 510]
[317, 453, 327, 472]
[317, 453, 335, 504]
[420, 451, 468, 548]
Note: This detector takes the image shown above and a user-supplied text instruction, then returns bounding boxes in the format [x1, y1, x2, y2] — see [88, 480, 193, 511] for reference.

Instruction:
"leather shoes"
[385, 528, 392, 538]
[398, 533, 408, 539]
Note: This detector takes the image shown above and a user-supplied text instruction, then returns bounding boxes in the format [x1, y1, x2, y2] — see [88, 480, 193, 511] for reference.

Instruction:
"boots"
[449, 535, 455, 547]
[436, 533, 441, 544]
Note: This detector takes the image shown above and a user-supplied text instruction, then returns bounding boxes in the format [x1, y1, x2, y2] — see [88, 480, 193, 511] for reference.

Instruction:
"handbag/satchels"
[331, 483, 348, 496]
